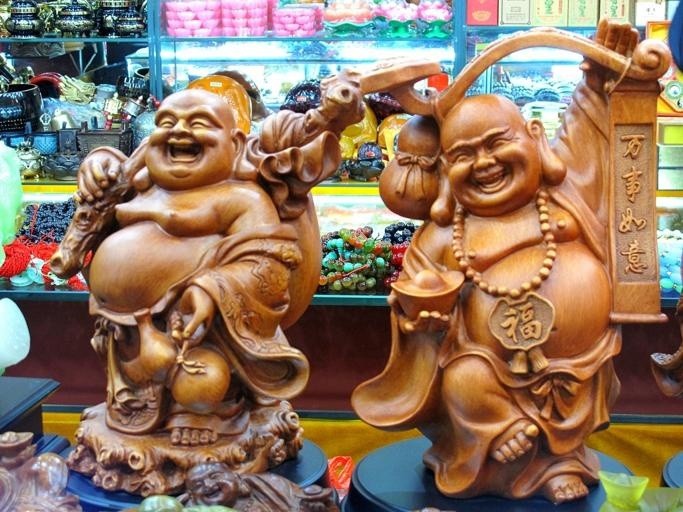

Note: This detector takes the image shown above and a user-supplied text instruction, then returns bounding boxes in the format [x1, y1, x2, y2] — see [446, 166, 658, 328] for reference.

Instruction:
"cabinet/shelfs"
[0, 0, 683, 423]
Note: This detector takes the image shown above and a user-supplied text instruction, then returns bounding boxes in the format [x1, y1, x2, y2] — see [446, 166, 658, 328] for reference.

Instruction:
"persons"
[349, 18, 641, 505]
[75, 89, 310, 448]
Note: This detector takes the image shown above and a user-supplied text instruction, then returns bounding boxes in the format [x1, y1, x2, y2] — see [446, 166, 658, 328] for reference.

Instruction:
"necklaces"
[447, 191, 558, 299]
